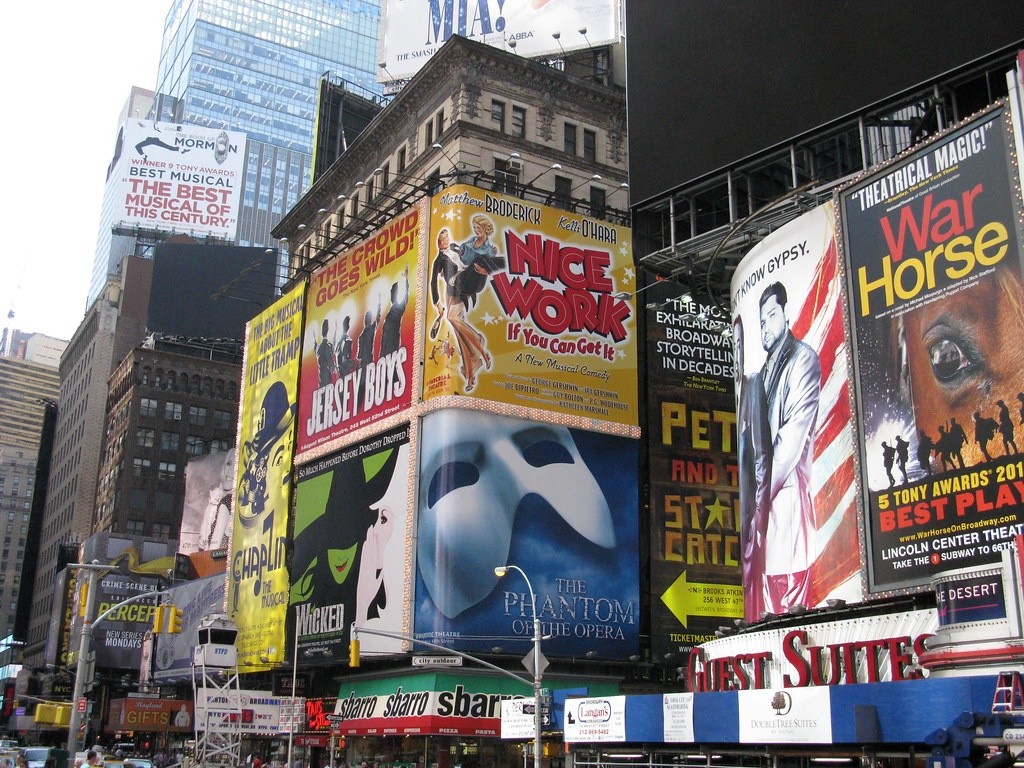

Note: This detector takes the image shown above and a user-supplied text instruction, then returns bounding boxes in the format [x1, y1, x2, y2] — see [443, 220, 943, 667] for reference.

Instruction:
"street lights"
[494, 565, 541, 767]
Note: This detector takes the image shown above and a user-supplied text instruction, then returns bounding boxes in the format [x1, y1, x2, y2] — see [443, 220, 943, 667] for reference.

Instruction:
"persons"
[0, 746, 380, 768]
[733, 280, 822, 623]
[199, 448, 236, 551]
[311, 263, 410, 425]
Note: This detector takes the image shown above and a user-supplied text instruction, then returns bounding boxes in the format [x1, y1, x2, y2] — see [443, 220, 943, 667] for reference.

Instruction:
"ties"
[764, 358, 774, 392]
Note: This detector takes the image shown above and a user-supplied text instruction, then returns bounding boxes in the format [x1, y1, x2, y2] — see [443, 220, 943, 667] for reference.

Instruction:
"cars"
[124, 759, 152, 768]
[20, 746, 52, 768]
